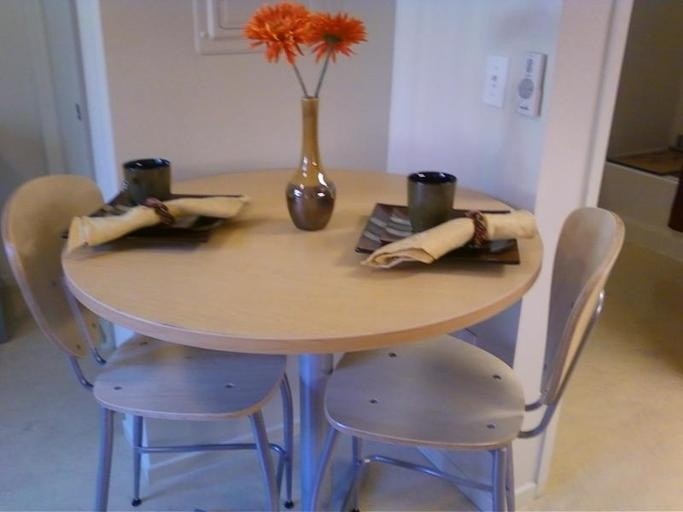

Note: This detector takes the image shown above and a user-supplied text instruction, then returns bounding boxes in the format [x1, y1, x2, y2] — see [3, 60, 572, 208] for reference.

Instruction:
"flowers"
[240, 2, 369, 100]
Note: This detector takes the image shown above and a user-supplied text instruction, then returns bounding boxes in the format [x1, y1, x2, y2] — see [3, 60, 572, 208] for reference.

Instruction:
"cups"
[122, 155, 173, 212]
[407, 169, 460, 238]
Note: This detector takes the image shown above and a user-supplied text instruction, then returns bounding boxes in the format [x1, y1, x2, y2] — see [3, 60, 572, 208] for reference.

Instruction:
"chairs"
[0, 171, 297, 511]
[308, 207, 625, 512]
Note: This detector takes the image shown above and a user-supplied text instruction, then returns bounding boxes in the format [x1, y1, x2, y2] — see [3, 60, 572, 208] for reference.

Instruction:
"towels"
[358, 209, 541, 271]
[65, 193, 249, 255]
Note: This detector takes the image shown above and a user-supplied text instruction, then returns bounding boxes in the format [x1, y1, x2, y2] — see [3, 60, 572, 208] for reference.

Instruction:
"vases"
[283, 97, 337, 232]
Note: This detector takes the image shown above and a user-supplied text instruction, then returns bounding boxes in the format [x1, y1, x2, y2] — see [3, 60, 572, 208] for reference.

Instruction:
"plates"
[55, 189, 248, 245]
[353, 199, 520, 272]
